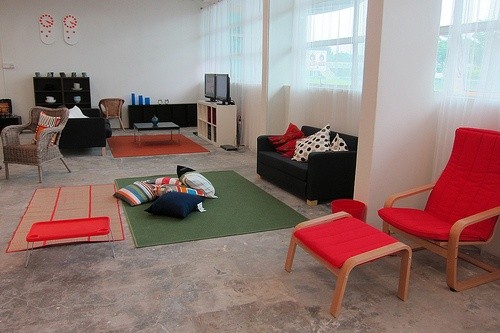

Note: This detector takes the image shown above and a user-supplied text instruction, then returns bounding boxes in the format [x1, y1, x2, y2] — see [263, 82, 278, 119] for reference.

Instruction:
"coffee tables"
[134, 122, 180, 147]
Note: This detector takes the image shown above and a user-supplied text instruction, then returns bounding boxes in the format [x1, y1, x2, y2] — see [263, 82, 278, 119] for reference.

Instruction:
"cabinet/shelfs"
[33, 77, 91, 109]
[128, 103, 197, 129]
[197, 100, 237, 148]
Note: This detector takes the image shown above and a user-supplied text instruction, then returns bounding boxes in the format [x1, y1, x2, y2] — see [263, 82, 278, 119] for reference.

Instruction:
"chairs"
[1, 106, 71, 183]
[99, 98, 125, 132]
[378, 127, 500, 291]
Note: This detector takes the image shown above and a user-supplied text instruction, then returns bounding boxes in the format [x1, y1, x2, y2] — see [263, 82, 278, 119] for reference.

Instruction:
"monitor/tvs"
[215, 74, 231, 104]
[205, 74, 216, 102]
[0, 99, 12, 118]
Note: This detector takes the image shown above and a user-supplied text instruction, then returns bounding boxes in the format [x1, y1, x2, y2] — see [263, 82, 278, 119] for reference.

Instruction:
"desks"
[25, 216, 115, 267]
[0, 116, 22, 134]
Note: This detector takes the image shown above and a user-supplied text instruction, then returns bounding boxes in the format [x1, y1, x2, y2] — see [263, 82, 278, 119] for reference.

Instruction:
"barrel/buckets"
[330, 199, 367, 222]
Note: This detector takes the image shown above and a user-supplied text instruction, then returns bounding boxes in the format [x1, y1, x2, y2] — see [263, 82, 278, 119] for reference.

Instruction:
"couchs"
[257, 126, 358, 206]
[59, 109, 112, 156]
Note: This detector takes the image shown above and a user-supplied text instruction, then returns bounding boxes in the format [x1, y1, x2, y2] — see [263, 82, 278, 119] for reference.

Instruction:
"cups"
[82, 73, 86, 77]
[158, 100, 162, 104]
[46, 97, 54, 102]
[74, 97, 81, 103]
[132, 93, 135, 105]
[139, 95, 143, 105]
[74, 83, 80, 89]
[72, 72, 76, 77]
[47, 72, 53, 77]
[165, 100, 169, 104]
[35, 72, 40, 77]
[145, 97, 150, 105]
[60, 72, 65, 77]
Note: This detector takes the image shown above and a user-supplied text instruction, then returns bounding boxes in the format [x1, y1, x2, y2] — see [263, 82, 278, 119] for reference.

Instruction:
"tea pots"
[152, 115, 159, 126]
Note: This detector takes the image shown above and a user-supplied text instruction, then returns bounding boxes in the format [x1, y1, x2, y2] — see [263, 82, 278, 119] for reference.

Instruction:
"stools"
[284, 211, 412, 318]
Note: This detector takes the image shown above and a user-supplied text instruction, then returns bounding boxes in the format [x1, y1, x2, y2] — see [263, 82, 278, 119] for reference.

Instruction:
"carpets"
[107, 134, 210, 158]
[6, 184, 124, 253]
[114, 169, 309, 248]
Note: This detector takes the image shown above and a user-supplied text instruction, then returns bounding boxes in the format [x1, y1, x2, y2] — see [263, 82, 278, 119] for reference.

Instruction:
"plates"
[72, 88, 82, 90]
[45, 100, 56, 103]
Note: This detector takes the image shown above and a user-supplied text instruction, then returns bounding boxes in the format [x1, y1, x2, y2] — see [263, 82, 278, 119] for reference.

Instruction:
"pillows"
[113, 164, 218, 219]
[267, 123, 349, 162]
[31, 111, 60, 146]
[68, 105, 89, 118]
